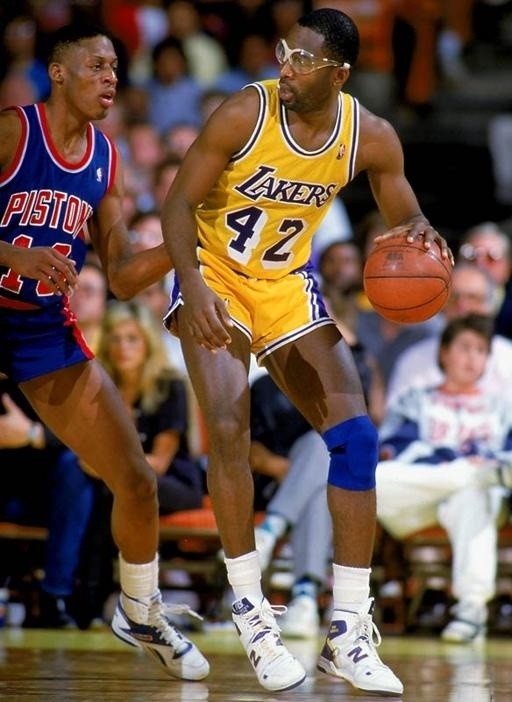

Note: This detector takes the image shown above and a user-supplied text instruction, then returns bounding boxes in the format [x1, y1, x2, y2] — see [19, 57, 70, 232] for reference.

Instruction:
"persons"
[159, 7, 456, 695]
[1, 1, 512, 639]
[1, 22, 213, 687]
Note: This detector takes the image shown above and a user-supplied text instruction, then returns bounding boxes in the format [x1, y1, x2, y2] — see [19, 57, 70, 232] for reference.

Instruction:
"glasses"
[273, 39, 341, 76]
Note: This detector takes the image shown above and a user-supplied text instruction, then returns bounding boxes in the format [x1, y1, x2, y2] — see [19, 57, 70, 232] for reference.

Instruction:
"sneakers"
[441, 604, 488, 644]
[218, 528, 274, 574]
[40, 597, 78, 630]
[277, 595, 319, 637]
[317, 597, 404, 696]
[230, 594, 306, 692]
[111, 591, 210, 681]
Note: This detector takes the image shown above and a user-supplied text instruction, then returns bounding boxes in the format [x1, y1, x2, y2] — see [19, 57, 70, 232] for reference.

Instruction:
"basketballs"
[364, 233, 454, 325]
